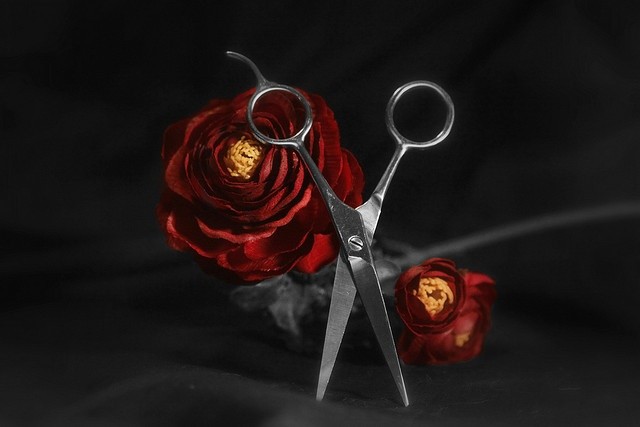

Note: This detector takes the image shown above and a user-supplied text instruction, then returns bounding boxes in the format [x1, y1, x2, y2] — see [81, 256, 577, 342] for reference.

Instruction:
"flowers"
[157, 85, 365, 284]
[395, 259, 494, 365]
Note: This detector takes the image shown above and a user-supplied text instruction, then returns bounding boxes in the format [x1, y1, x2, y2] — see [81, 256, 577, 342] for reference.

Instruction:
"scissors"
[224, 49, 455, 407]
